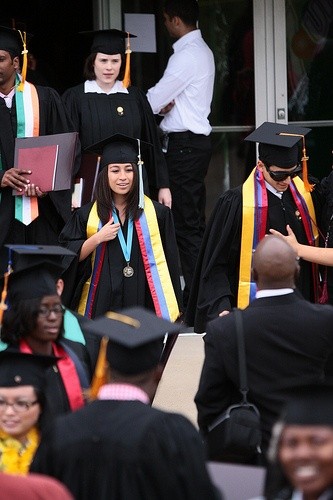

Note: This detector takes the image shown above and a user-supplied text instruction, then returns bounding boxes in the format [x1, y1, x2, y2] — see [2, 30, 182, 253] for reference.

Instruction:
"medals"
[122, 258, 136, 278]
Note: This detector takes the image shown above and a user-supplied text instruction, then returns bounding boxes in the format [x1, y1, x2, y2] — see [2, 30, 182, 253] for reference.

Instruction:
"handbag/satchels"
[203, 403, 269, 465]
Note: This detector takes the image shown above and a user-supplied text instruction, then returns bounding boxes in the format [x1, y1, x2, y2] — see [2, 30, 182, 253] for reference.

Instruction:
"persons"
[262, 224, 333, 266]
[63, 29, 173, 210]
[26, 305, 226, 500]
[59, 133, 185, 325]
[262, 371, 333, 500]
[193, 232, 333, 465]
[0, 26, 71, 244]
[0, 470, 76, 500]
[0, 349, 67, 477]
[0, 242, 103, 442]
[186, 121, 326, 332]
[145, 0, 216, 305]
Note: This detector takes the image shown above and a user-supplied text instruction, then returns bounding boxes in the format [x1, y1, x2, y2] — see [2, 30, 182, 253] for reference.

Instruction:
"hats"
[270, 376, 333, 426]
[3, 243, 78, 271]
[0, 25, 34, 91]
[81, 306, 185, 403]
[244, 121, 316, 193]
[86, 133, 154, 210]
[81, 28, 138, 89]
[6, 258, 64, 298]
[0, 350, 64, 389]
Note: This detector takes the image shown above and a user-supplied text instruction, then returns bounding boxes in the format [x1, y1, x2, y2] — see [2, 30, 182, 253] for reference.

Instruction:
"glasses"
[39, 304, 66, 318]
[0, 399, 39, 413]
[264, 165, 302, 182]
